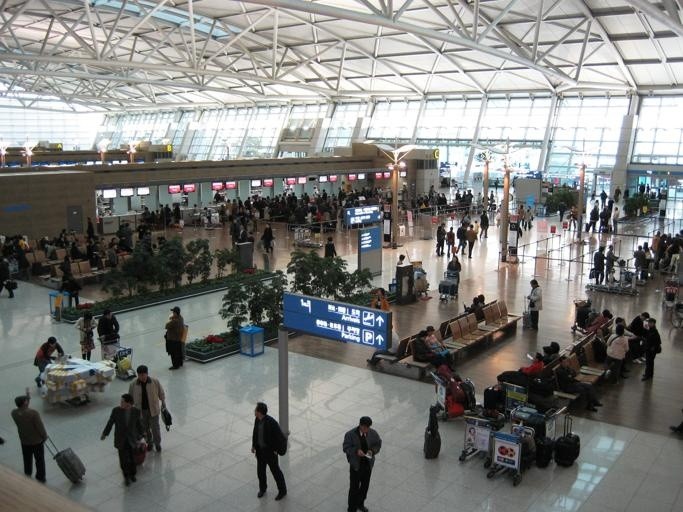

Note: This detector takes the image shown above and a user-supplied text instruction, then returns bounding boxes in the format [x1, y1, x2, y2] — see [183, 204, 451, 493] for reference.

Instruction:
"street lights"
[566, 146, 592, 242]
[488, 146, 516, 261]
[471, 143, 496, 212]
[376, 143, 419, 247]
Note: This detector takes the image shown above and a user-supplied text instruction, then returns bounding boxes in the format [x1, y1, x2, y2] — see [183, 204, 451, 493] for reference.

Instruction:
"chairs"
[78, 260, 90, 272]
[34, 250, 43, 262]
[458, 317, 484, 342]
[71, 263, 79, 274]
[482, 306, 506, 330]
[497, 299, 521, 322]
[449, 320, 476, 346]
[56, 248, 67, 259]
[490, 303, 514, 327]
[25, 253, 35, 264]
[467, 313, 492, 337]
[581, 342, 606, 369]
[569, 353, 598, 384]
[54, 265, 64, 277]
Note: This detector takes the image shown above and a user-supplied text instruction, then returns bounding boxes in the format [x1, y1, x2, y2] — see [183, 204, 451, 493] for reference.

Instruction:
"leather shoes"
[258, 488, 267, 498]
[131, 476, 136, 481]
[593, 401, 603, 406]
[126, 479, 129, 486]
[359, 506, 368, 512]
[275, 490, 287, 500]
[641, 375, 649, 381]
[586, 405, 597, 412]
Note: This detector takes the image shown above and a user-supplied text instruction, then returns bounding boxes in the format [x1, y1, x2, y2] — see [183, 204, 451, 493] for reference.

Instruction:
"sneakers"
[641, 357, 646, 361]
[633, 359, 642, 363]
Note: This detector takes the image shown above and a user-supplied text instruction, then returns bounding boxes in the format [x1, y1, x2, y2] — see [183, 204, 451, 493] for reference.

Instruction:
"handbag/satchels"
[438, 365, 476, 416]
[160, 406, 172, 431]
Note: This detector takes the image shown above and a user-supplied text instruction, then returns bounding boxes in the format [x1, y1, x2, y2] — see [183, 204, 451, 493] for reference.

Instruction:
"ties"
[362, 433, 365, 440]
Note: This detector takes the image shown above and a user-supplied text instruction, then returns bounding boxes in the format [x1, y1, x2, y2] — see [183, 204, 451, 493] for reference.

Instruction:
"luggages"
[566, 416, 580, 459]
[554, 413, 575, 466]
[536, 437, 554, 468]
[44, 434, 86, 482]
[523, 295, 531, 327]
[483, 385, 505, 413]
[133, 440, 146, 466]
[424, 404, 441, 459]
[527, 409, 555, 440]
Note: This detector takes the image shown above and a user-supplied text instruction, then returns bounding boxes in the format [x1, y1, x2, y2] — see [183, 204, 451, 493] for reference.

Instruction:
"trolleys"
[440, 271, 458, 303]
[459, 416, 493, 468]
[571, 300, 589, 334]
[662, 286, 679, 312]
[41, 357, 90, 410]
[487, 431, 521, 486]
[585, 269, 640, 297]
[430, 371, 482, 422]
[97, 334, 137, 380]
[669, 299, 683, 328]
[293, 228, 324, 248]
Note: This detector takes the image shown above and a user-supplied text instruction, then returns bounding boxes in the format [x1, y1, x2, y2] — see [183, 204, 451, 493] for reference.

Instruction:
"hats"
[646, 318, 656, 323]
[418, 330, 428, 337]
[170, 307, 180, 314]
[427, 326, 435, 331]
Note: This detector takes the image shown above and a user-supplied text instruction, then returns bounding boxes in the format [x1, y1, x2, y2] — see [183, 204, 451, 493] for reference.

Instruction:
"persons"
[435, 361, 453, 393]
[599, 323, 629, 386]
[612, 316, 638, 379]
[643, 243, 650, 254]
[1, 195, 181, 309]
[478, 294, 485, 307]
[634, 246, 645, 279]
[519, 353, 544, 375]
[599, 209, 616, 233]
[669, 421, 683, 435]
[528, 280, 542, 330]
[11, 396, 47, 484]
[606, 245, 619, 282]
[568, 206, 577, 232]
[465, 297, 480, 315]
[591, 191, 596, 200]
[543, 347, 553, 363]
[372, 290, 388, 310]
[640, 183, 645, 194]
[608, 198, 614, 216]
[641, 318, 661, 381]
[100, 394, 146, 487]
[448, 255, 461, 294]
[523, 206, 533, 230]
[646, 184, 649, 193]
[367, 324, 398, 364]
[398, 255, 406, 270]
[192, 178, 500, 257]
[413, 331, 444, 368]
[129, 365, 166, 452]
[34, 336, 64, 388]
[559, 199, 567, 222]
[601, 191, 607, 208]
[587, 205, 599, 233]
[623, 186, 629, 200]
[551, 341, 561, 361]
[628, 312, 650, 364]
[426, 326, 456, 372]
[601, 309, 610, 324]
[343, 416, 382, 512]
[594, 246, 604, 284]
[74, 311, 98, 361]
[518, 205, 524, 226]
[614, 186, 621, 204]
[650, 230, 683, 270]
[97, 310, 120, 338]
[164, 306, 184, 370]
[251, 402, 288, 501]
[556, 356, 604, 413]
